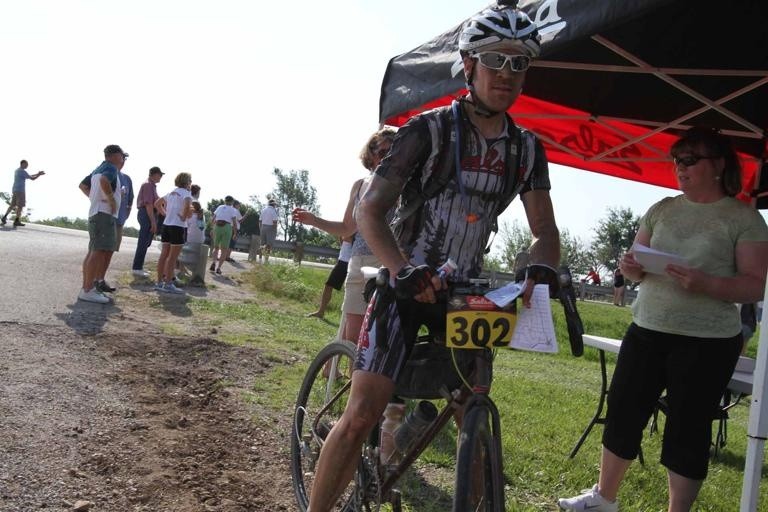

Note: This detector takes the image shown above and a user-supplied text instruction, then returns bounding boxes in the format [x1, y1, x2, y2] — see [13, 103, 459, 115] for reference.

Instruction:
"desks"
[568, 331, 755, 471]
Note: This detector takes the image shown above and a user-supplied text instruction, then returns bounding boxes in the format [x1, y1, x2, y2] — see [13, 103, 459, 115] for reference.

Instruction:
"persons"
[292, 131, 400, 385]
[307, 5, 560, 512]
[558, 125, 768, 512]
[513, 244, 529, 270]
[585, 268, 600, 285]
[309, 235, 354, 317]
[322, 322, 346, 378]
[78, 146, 278, 304]
[1, 160, 46, 226]
[613, 269, 625, 306]
[740, 301, 758, 357]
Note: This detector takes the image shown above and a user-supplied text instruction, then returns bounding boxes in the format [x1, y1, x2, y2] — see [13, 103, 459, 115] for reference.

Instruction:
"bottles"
[380, 399, 409, 468]
[393, 398, 438, 450]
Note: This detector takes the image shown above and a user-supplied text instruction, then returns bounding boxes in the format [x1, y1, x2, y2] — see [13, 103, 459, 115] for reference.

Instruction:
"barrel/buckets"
[176, 244, 210, 285]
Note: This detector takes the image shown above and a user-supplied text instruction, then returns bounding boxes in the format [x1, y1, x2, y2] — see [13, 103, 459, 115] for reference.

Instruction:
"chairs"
[648, 301, 755, 454]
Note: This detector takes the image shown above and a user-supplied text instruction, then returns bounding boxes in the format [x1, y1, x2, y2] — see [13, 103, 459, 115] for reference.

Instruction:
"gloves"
[394, 262, 444, 302]
[513, 264, 561, 296]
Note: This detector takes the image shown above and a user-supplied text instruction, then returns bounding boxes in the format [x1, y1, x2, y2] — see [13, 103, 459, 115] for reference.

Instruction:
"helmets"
[458, 5, 542, 58]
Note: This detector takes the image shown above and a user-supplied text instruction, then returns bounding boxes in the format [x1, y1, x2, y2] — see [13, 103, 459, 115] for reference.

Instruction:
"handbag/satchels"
[216, 219, 226, 227]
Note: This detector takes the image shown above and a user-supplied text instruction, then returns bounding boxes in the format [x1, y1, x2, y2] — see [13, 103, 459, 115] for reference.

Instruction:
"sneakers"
[132, 268, 151, 278]
[154, 282, 174, 292]
[79, 286, 109, 304]
[1, 215, 8, 224]
[13, 220, 26, 226]
[172, 276, 188, 288]
[216, 268, 222, 274]
[209, 262, 216, 271]
[95, 280, 117, 292]
[559, 483, 619, 511]
[225, 258, 235, 262]
[161, 281, 185, 294]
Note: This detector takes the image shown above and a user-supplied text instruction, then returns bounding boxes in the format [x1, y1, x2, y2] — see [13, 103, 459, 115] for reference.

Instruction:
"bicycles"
[287, 262, 587, 511]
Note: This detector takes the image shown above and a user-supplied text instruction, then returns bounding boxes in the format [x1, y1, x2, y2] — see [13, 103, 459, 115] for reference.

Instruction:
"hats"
[148, 167, 166, 175]
[225, 195, 234, 200]
[103, 144, 130, 157]
[268, 199, 277, 204]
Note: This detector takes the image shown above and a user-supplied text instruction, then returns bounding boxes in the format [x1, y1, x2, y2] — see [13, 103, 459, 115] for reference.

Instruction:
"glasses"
[470, 50, 532, 73]
[374, 148, 391, 157]
[673, 153, 712, 168]
